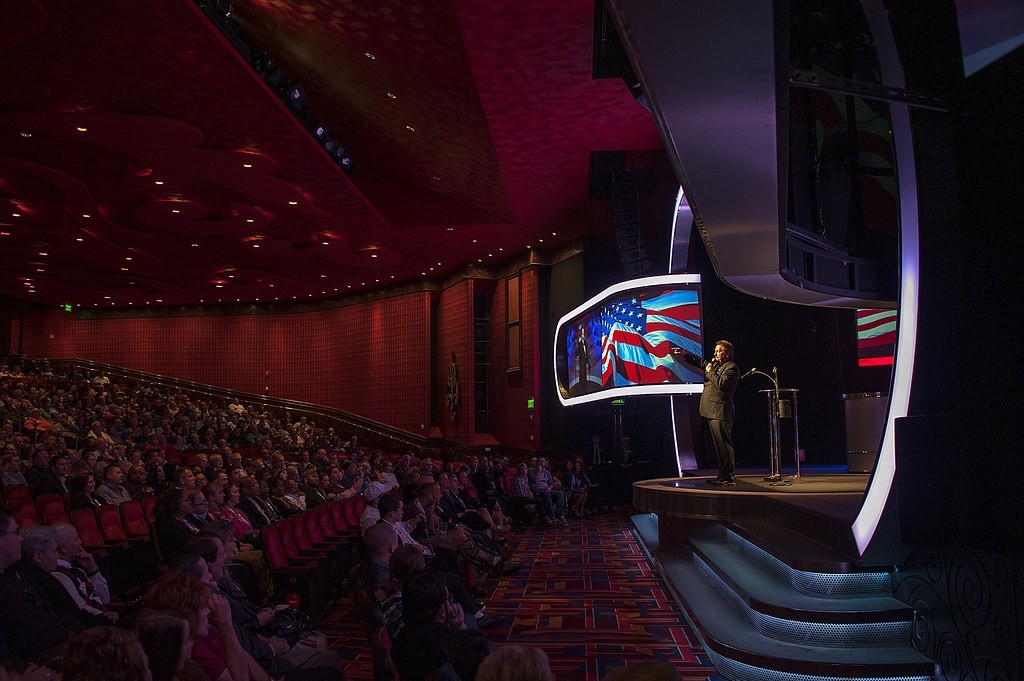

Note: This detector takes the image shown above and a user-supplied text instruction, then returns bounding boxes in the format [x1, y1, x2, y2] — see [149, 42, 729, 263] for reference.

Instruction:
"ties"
[594, 445, 599, 465]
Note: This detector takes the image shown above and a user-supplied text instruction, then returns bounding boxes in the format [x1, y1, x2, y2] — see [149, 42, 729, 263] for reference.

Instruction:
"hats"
[342, 460, 352, 471]
[303, 469, 319, 476]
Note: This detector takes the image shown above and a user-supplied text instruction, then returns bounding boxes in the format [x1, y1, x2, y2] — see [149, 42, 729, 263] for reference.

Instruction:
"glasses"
[192, 498, 207, 506]
[175, 497, 190, 503]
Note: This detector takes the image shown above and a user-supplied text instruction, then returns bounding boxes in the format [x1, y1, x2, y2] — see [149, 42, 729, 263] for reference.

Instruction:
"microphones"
[704, 358, 716, 373]
[741, 368, 756, 379]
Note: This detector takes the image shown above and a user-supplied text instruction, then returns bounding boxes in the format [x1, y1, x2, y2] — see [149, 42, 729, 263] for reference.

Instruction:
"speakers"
[611, 168, 652, 280]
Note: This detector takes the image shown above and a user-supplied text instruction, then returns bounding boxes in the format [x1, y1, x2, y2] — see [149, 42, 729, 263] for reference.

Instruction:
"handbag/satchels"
[265, 608, 312, 639]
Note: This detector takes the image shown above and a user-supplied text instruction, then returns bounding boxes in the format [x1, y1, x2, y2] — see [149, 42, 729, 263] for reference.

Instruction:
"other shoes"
[470, 503, 619, 629]
[706, 475, 723, 483]
[711, 478, 736, 487]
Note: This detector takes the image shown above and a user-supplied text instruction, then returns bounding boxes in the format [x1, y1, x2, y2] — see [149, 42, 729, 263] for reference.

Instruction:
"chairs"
[0, 446, 564, 681]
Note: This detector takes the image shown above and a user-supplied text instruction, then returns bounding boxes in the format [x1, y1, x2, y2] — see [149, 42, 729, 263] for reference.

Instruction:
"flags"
[601, 290, 704, 391]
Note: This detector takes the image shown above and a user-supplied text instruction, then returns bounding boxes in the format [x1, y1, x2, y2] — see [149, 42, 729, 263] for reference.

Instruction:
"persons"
[364, 494, 496, 681]
[0, 351, 358, 453]
[584, 434, 608, 510]
[575, 324, 590, 395]
[369, 447, 519, 595]
[0, 504, 131, 681]
[601, 661, 682, 681]
[671, 340, 740, 485]
[475, 644, 552, 681]
[131, 532, 345, 681]
[0, 453, 168, 532]
[512, 456, 599, 527]
[611, 435, 639, 506]
[153, 453, 371, 606]
